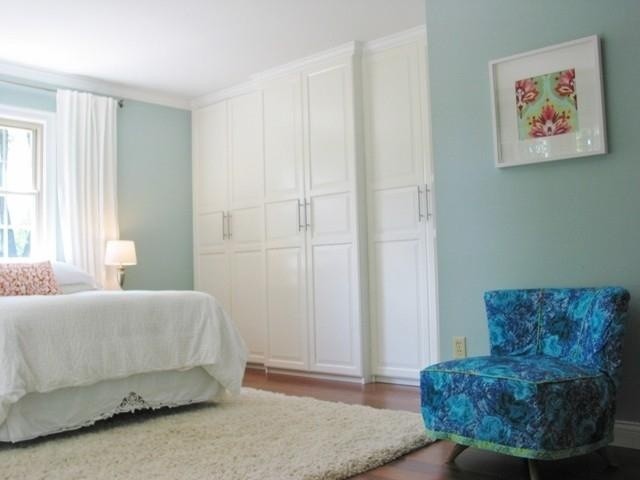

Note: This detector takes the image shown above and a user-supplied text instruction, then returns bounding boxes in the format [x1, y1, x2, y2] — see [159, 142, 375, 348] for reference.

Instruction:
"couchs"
[421, 286, 630, 480]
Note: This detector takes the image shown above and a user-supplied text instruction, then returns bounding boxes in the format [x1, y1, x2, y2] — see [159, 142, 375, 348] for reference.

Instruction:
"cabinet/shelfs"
[265, 38, 363, 380]
[364, 22, 440, 386]
[190, 70, 264, 371]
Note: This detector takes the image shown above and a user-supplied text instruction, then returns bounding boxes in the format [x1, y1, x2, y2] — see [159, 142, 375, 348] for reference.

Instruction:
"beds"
[0, 265, 249, 444]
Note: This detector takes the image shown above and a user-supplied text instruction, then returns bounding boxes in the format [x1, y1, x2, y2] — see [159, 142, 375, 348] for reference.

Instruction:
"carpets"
[0, 386, 441, 480]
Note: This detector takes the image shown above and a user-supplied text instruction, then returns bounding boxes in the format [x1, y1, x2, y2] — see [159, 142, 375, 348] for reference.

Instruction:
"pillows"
[0, 260, 60, 296]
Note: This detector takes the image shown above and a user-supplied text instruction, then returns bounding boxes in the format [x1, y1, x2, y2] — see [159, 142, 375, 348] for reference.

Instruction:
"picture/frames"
[488, 34, 608, 168]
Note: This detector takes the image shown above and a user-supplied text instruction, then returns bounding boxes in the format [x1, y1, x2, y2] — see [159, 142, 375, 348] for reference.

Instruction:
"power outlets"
[454, 337, 465, 358]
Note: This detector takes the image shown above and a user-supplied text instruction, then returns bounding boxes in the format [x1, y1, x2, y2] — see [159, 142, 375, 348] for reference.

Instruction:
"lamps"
[103, 240, 137, 287]
